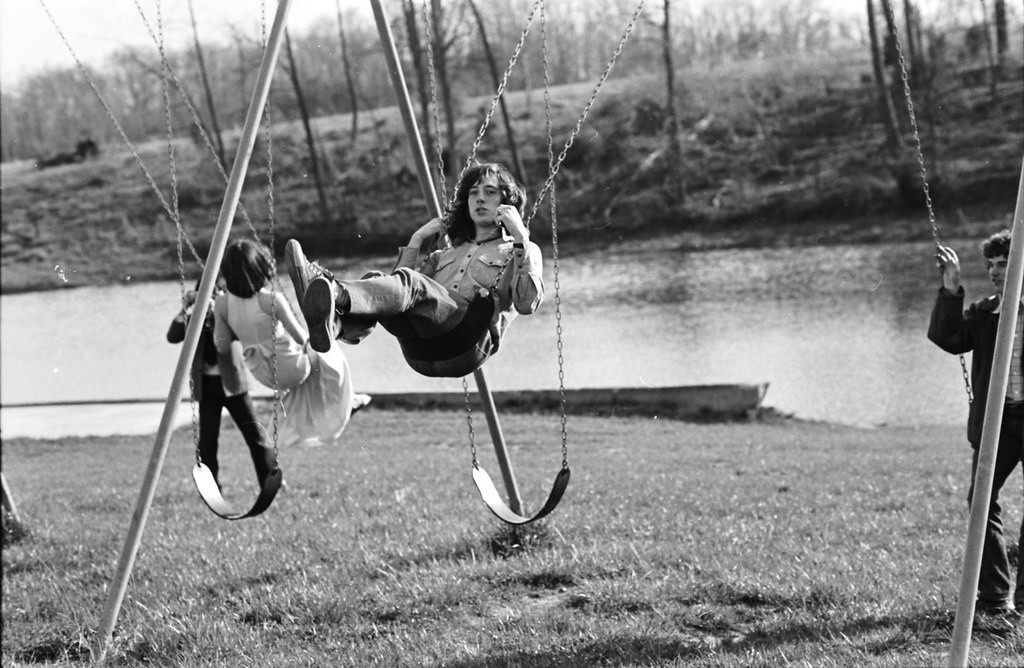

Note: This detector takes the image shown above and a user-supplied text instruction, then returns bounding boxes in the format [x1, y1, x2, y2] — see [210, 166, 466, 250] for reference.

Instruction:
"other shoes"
[351, 394, 372, 414]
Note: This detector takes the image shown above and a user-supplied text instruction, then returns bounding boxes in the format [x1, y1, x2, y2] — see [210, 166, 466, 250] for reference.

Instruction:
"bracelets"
[515, 240, 528, 250]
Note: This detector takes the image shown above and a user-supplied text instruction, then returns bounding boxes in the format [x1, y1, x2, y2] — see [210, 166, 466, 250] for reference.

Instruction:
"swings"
[395, 0, 648, 363]
[37, 3, 321, 380]
[157, 1, 284, 523]
[419, 1, 572, 526]
[881, 0, 976, 406]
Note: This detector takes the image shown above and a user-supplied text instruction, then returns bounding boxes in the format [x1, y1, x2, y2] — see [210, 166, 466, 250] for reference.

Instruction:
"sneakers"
[285, 238, 334, 309]
[301, 276, 338, 353]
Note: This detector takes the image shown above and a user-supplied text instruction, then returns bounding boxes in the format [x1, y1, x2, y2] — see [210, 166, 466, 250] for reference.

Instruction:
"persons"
[928, 230, 1024, 613]
[283, 162, 545, 378]
[214, 239, 371, 418]
[167, 275, 290, 495]
